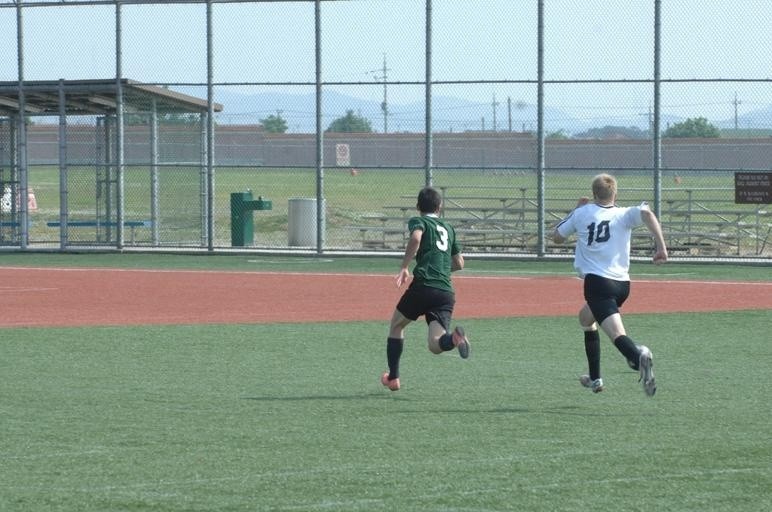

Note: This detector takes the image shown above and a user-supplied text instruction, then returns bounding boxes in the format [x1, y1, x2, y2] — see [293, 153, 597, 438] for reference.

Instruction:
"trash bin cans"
[288, 196, 326, 248]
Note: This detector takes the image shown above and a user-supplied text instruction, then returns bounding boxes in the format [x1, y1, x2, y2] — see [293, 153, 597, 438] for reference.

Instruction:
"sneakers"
[579, 373, 604, 393]
[637, 345, 656, 398]
[450, 325, 472, 359]
[381, 372, 400, 392]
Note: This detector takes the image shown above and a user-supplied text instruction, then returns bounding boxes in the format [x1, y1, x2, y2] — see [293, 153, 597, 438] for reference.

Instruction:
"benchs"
[46, 220, 153, 246]
[0, 221, 37, 246]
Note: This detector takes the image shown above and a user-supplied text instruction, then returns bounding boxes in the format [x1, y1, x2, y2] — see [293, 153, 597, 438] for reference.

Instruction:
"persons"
[1, 184, 38, 237]
[553, 172, 668, 398]
[382, 185, 472, 391]
